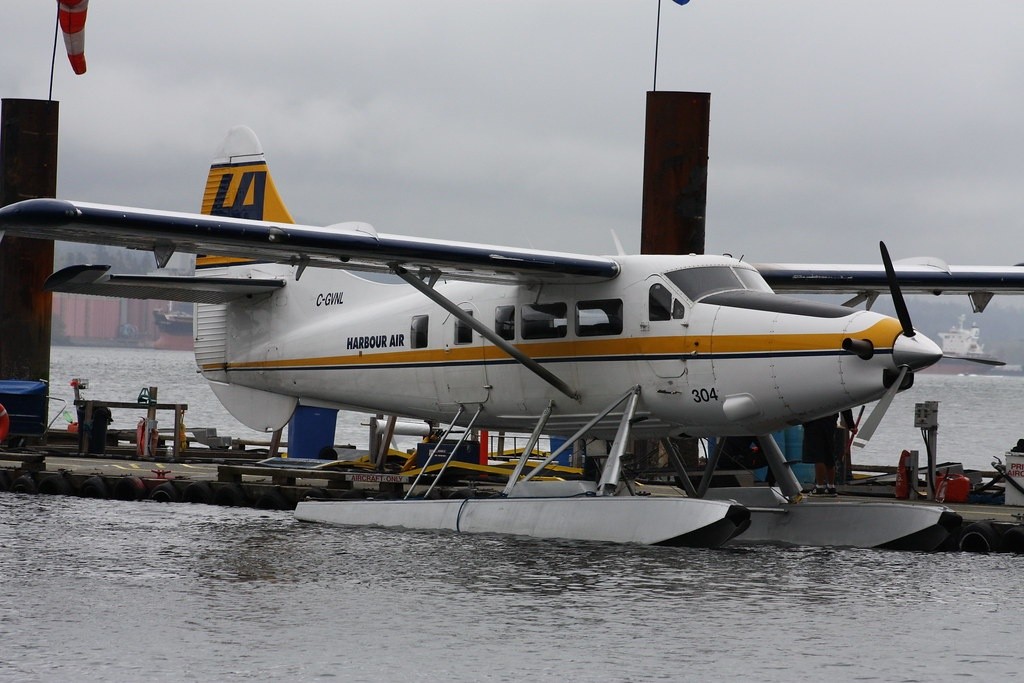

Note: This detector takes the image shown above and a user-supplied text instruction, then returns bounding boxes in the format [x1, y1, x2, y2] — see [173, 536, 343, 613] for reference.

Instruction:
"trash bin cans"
[76, 407, 108, 453]
[286, 405, 340, 461]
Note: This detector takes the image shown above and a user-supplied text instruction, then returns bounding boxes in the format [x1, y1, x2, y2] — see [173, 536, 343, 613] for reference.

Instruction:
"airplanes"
[0, 124, 1024, 554]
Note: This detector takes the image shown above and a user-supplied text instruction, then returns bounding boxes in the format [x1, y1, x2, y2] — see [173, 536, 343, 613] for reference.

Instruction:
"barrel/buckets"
[934, 471, 971, 502]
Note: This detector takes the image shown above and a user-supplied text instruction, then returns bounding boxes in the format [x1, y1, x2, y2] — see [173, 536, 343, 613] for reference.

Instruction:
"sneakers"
[801, 485, 825, 496]
[824, 488, 837, 496]
